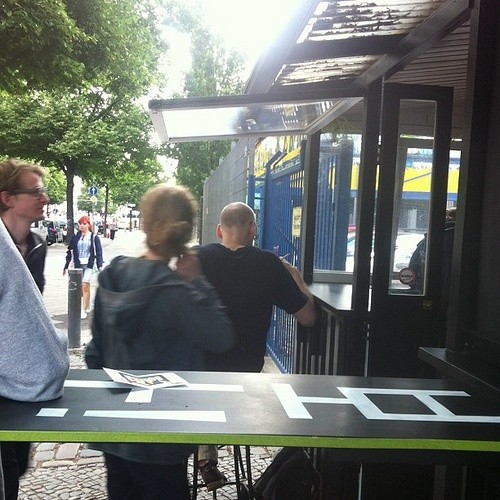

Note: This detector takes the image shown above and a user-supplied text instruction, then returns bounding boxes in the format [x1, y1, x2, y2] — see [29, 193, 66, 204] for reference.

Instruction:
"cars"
[30, 219, 78, 245]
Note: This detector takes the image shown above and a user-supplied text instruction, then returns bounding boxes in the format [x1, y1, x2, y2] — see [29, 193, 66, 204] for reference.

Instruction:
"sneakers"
[199, 461, 227, 491]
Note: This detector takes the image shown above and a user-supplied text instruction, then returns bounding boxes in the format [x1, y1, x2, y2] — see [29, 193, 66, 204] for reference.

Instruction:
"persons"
[62, 217, 103, 319]
[54, 224, 67, 243]
[191, 202, 316, 491]
[103, 218, 118, 239]
[85, 184, 237, 500]
[0, 161, 71, 500]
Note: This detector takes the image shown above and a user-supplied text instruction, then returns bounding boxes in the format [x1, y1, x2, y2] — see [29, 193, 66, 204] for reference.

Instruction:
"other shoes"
[81, 312, 87, 319]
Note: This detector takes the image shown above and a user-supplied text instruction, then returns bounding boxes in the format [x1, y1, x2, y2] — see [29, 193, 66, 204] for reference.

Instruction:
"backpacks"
[253, 446, 320, 500]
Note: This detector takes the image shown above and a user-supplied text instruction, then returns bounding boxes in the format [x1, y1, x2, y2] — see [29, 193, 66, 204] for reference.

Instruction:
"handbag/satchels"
[91, 263, 100, 287]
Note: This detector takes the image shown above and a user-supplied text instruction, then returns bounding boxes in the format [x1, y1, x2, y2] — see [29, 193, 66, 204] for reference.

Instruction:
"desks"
[0, 369, 500, 500]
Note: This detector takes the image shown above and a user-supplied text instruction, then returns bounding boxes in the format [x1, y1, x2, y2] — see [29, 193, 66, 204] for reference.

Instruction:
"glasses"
[12, 187, 44, 196]
[253, 222, 257, 240]
